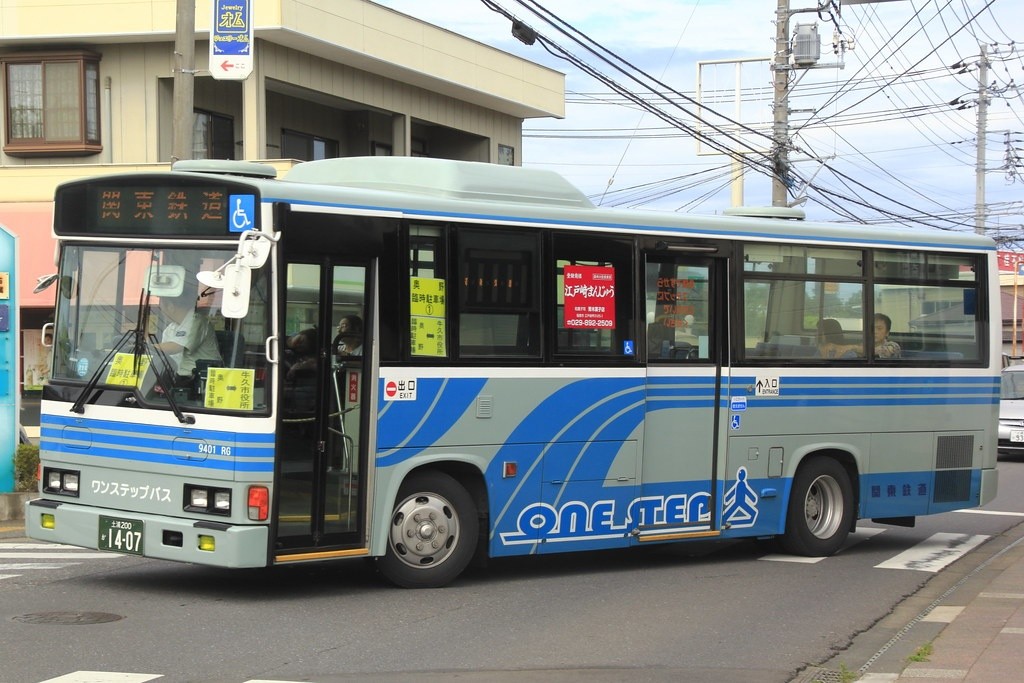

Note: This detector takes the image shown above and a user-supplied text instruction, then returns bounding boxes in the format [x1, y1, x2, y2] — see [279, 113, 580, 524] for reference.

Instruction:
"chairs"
[674, 341, 964, 360]
[214, 330, 245, 369]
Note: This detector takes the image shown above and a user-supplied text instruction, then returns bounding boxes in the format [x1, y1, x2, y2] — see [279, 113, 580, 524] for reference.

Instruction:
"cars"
[997, 365, 1024, 453]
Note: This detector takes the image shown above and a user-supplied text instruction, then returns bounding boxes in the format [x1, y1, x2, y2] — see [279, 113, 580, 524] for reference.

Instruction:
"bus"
[24, 159, 1004, 589]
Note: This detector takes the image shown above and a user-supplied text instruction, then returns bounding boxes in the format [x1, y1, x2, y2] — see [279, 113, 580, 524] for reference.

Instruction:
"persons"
[816, 319, 843, 345]
[150, 291, 225, 401]
[819, 312, 902, 358]
[284, 328, 318, 380]
[337, 315, 363, 356]
[40, 333, 54, 378]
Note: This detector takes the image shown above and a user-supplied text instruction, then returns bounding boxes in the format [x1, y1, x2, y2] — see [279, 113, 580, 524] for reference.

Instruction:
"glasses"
[337, 323, 352, 329]
